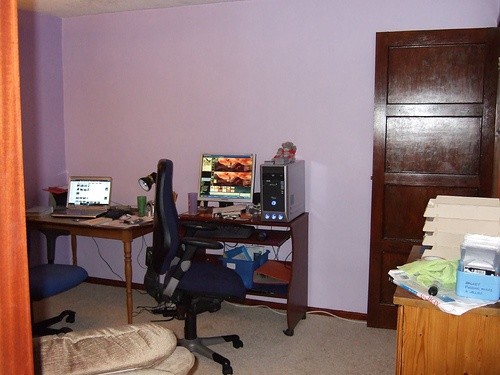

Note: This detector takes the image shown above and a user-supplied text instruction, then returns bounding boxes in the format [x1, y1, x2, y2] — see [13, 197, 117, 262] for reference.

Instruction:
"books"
[393, 277, 455, 303]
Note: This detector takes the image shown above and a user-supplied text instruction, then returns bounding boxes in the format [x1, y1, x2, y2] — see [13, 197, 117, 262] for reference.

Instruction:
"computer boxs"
[260, 159, 305, 221]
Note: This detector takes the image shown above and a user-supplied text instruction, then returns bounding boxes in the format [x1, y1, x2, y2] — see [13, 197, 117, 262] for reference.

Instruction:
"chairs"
[144, 158, 244, 375]
[27, 224, 88, 335]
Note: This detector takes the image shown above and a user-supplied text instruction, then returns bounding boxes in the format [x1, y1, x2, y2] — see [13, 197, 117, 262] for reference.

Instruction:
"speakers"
[187, 192, 198, 215]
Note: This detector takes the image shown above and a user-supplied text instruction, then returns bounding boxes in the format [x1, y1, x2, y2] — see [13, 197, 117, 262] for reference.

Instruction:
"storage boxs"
[217, 250, 270, 289]
[455, 259, 500, 301]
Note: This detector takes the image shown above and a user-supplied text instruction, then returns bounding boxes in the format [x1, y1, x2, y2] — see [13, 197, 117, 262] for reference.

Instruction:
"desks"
[25, 212, 154, 325]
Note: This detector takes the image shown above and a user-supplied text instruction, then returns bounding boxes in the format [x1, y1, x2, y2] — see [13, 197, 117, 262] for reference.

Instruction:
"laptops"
[51, 176, 112, 218]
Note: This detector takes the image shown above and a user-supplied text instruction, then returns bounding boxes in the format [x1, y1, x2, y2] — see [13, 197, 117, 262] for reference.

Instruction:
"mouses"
[257, 233, 267, 241]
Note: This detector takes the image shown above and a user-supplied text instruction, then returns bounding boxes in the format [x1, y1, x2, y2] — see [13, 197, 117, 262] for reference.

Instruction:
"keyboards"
[193, 222, 256, 239]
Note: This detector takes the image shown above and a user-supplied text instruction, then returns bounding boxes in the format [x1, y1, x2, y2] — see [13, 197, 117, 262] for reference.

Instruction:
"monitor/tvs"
[197, 153, 257, 208]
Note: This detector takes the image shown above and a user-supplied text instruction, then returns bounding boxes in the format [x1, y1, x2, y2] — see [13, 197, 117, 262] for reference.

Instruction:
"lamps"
[139, 172, 158, 192]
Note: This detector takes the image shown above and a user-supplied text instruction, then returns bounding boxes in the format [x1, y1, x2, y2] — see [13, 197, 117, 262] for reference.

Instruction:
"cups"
[137, 196, 146, 217]
[188, 193, 198, 214]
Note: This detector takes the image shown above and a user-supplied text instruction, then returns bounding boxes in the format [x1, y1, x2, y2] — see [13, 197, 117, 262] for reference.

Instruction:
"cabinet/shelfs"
[177, 207, 309, 336]
[393, 245, 500, 375]
